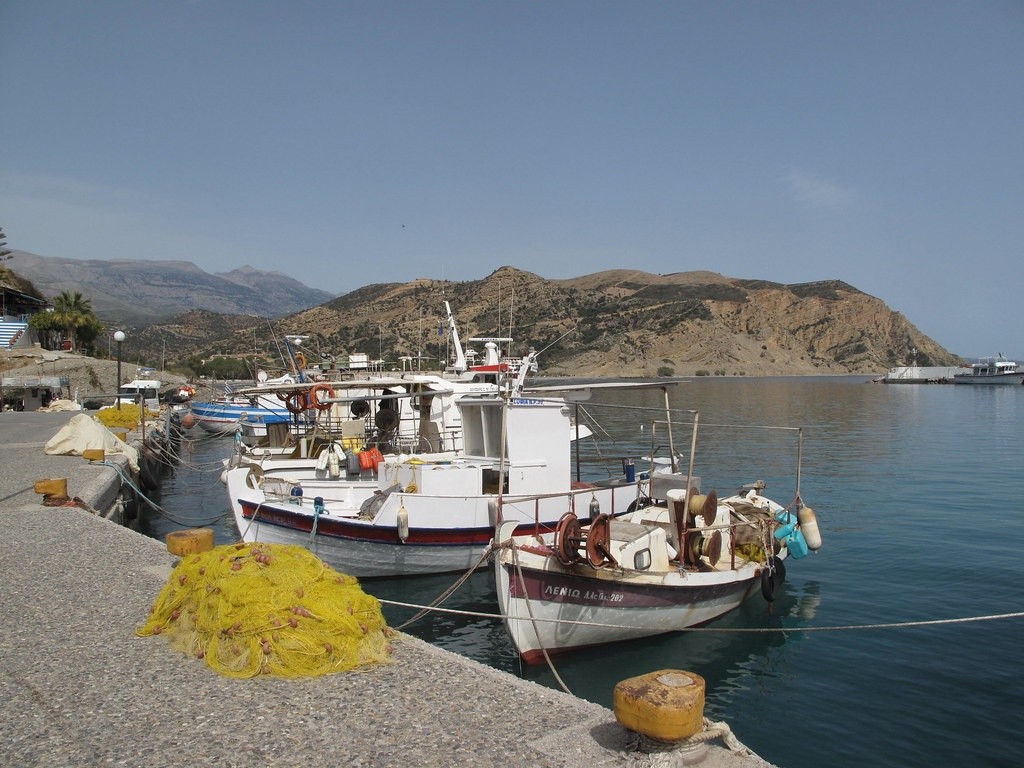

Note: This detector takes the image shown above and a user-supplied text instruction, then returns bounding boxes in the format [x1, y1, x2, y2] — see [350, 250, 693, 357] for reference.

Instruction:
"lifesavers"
[309, 385, 336, 411]
[285, 390, 308, 414]
[351, 401, 369, 417]
[290, 354, 307, 370]
[374, 408, 399, 432]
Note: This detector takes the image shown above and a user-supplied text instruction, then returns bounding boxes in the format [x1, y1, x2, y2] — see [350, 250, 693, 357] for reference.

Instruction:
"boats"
[226, 283, 700, 580]
[113, 300, 593, 462]
[493, 385, 803, 668]
[953, 352, 1024, 386]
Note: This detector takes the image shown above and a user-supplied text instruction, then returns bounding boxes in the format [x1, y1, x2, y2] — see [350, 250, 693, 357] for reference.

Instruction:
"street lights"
[113, 331, 126, 411]
